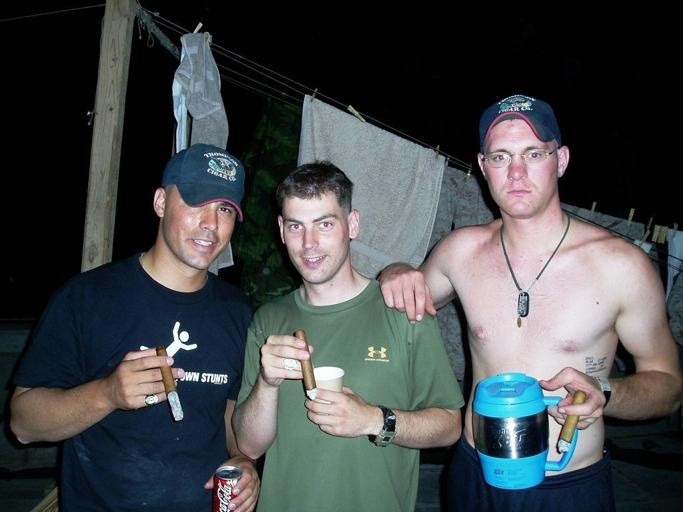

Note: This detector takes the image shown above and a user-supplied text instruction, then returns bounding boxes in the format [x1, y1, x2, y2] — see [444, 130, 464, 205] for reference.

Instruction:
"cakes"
[212, 465, 242, 512]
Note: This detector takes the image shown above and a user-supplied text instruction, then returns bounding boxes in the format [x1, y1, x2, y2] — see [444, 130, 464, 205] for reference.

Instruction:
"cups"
[483, 146, 559, 168]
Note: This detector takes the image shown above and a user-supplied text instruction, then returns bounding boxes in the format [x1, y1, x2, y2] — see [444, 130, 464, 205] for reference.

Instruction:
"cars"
[311, 367, 345, 404]
[472, 372, 578, 491]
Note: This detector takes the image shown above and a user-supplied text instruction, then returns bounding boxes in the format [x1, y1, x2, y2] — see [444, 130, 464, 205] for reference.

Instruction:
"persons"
[378, 92, 683, 510]
[3, 141, 261, 512]
[231, 159, 468, 512]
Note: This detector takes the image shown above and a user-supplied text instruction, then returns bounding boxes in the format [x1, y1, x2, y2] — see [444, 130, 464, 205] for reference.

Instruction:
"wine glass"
[144, 394, 159, 405]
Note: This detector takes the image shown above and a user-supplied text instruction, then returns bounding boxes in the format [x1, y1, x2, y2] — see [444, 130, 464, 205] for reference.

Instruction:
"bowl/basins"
[497, 207, 571, 319]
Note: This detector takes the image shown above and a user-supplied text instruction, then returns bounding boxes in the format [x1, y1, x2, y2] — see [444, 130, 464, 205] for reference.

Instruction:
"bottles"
[479, 94, 561, 144]
[162, 144, 245, 222]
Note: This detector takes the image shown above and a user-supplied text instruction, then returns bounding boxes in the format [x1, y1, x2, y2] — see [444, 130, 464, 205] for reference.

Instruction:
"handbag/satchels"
[595, 375, 613, 406]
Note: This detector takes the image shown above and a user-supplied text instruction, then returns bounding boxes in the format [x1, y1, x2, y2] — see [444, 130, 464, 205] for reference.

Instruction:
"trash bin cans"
[369, 403, 398, 451]
